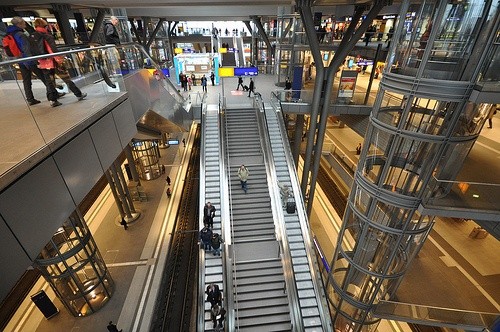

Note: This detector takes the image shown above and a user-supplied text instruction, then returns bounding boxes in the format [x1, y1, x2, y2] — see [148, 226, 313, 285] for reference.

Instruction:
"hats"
[11, 17, 25, 24]
[34, 18, 48, 27]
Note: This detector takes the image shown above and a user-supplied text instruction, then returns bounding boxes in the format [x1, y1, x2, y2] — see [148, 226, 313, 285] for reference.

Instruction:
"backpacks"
[28, 32, 46, 60]
[1, 31, 24, 57]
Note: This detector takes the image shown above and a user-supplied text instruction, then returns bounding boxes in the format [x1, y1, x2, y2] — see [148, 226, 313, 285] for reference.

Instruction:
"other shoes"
[28, 99, 41, 105]
[50, 100, 62, 106]
[77, 93, 87, 100]
[56, 92, 65, 98]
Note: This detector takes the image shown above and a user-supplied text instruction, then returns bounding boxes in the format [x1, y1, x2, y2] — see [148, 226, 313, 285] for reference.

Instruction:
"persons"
[198, 227, 213, 250]
[107, 321, 122, 332]
[318, 20, 395, 46]
[356, 143, 361, 155]
[236, 75, 244, 91]
[207, 283, 220, 306]
[104, 16, 129, 70]
[201, 74, 208, 93]
[210, 304, 224, 328]
[7, 16, 66, 106]
[209, 233, 225, 256]
[308, 65, 312, 80]
[284, 77, 292, 90]
[204, 202, 216, 228]
[121, 217, 128, 230]
[237, 164, 250, 193]
[182, 138, 186, 147]
[179, 71, 196, 91]
[166, 176, 171, 185]
[33, 18, 88, 107]
[247, 78, 255, 97]
[210, 72, 215, 86]
[51, 25, 91, 41]
[166, 188, 172, 198]
[161, 164, 165, 174]
[486, 104, 497, 129]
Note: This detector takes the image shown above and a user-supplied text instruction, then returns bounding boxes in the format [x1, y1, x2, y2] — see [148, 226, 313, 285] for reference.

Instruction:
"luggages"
[243, 85, 249, 91]
[254, 92, 261, 97]
[287, 195, 296, 213]
